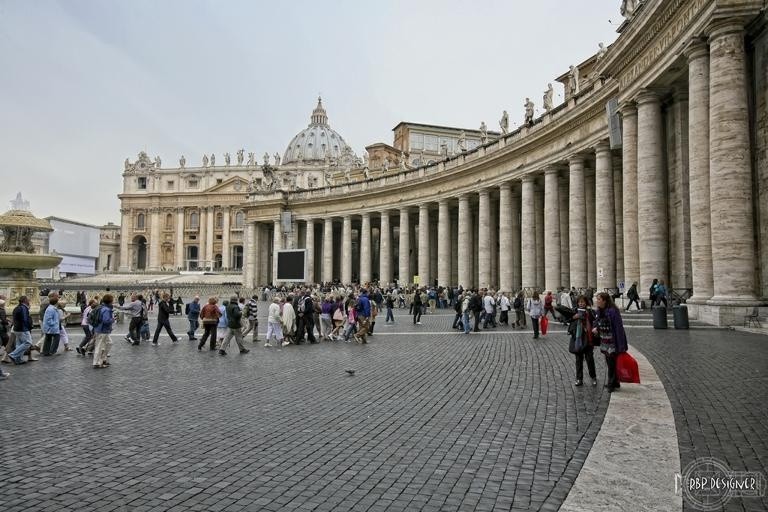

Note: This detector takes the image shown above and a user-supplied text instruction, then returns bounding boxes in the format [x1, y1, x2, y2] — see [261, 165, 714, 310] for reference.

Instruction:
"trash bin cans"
[652, 306, 667, 329]
[673, 305, 689, 329]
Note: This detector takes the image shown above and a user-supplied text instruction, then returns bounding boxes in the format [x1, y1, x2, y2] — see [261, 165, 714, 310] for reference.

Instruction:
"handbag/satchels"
[569, 338, 584, 354]
[593, 333, 601, 345]
[333, 309, 343, 321]
[617, 352, 640, 383]
[373, 308, 377, 317]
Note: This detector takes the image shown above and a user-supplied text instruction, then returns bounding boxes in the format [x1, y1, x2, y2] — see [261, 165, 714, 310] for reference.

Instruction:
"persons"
[0, 275, 669, 394]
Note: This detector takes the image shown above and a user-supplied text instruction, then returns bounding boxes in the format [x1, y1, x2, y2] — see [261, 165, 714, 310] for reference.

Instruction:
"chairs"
[744, 307, 763, 329]
[425, 299, 437, 315]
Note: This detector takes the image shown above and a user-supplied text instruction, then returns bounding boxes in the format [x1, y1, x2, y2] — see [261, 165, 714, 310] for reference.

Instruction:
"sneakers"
[2, 344, 110, 369]
[185, 330, 373, 356]
[605, 384, 619, 389]
[452, 321, 539, 339]
[0, 372, 10, 379]
[124, 335, 152, 345]
[593, 380, 597, 386]
[172, 338, 182, 343]
[417, 322, 422, 325]
[625, 310, 631, 313]
[575, 380, 583, 385]
[608, 386, 615, 393]
[638, 309, 644, 313]
[151, 343, 161, 347]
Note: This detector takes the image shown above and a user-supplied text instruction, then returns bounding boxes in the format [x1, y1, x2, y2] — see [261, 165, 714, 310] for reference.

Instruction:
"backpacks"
[87, 304, 104, 328]
[297, 296, 310, 312]
[515, 297, 521, 307]
[242, 304, 251, 318]
[454, 296, 464, 312]
[354, 298, 364, 311]
